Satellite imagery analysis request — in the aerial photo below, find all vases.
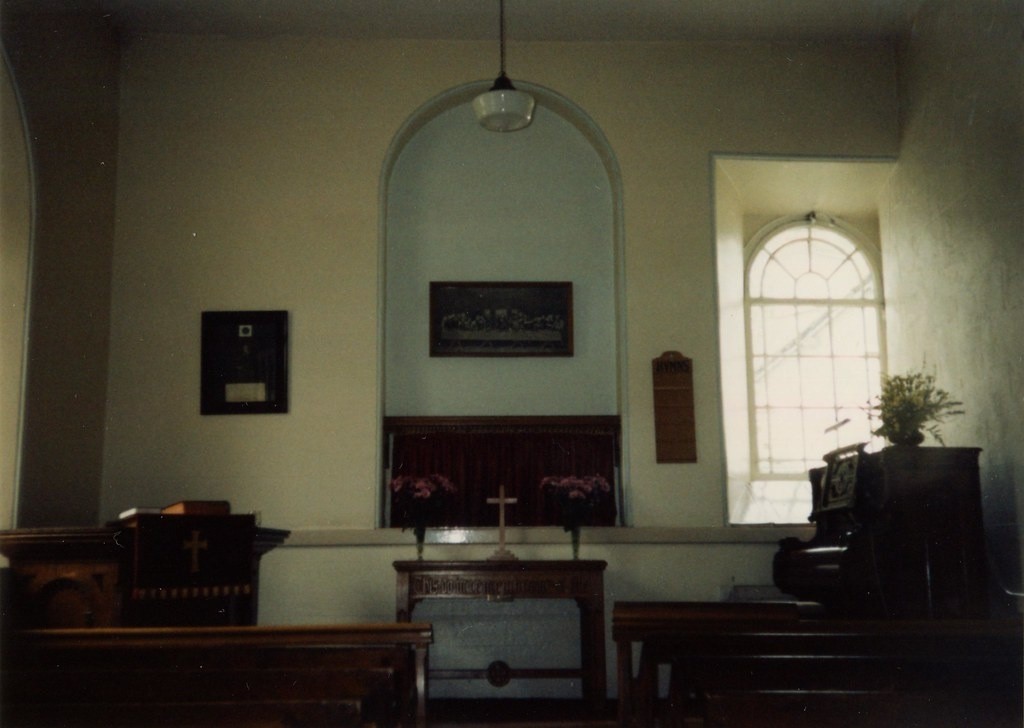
[416,527,425,560]
[571,526,581,559]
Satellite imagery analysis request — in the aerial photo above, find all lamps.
[473,0,535,134]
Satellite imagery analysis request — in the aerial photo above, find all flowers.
[387,473,463,541]
[539,474,609,548]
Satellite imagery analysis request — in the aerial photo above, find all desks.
[811,446,992,621]
[393,559,609,720]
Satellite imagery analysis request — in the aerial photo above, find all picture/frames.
[199,308,289,415]
[429,280,574,358]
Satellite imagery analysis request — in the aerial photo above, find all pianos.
[772,445,989,620]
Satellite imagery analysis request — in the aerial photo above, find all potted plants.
[863,370,966,447]
